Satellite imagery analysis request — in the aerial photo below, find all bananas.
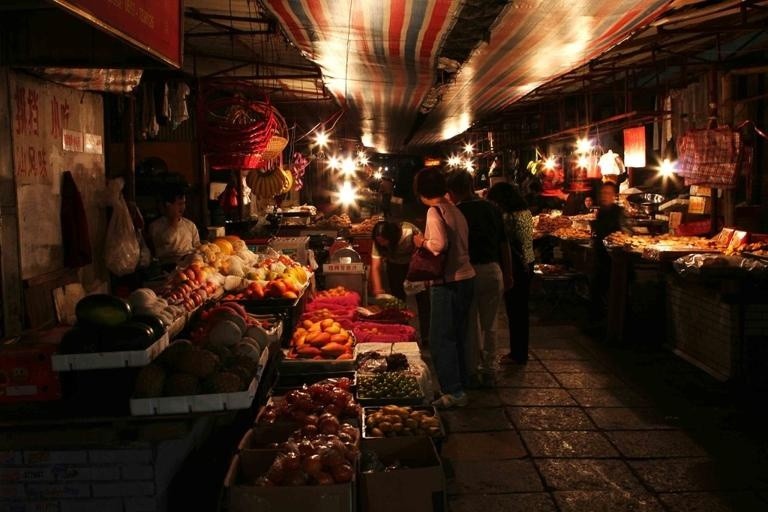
[246,167,291,200]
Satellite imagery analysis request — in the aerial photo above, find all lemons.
[251,378,441,488]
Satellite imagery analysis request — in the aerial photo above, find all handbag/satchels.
[676,117,742,187]
[405,205,445,282]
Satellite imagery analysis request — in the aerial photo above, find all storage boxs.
[222,405,448,512]
[0,326,69,404]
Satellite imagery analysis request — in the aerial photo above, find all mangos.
[289,317,352,359]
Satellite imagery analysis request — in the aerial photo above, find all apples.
[151,264,215,312]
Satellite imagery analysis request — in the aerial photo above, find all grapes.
[354,342,409,372]
[362,296,408,324]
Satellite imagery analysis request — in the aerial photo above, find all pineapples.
[133,338,256,398]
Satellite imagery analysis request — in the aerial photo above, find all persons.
[489,183,537,364]
[378,177,394,203]
[579,181,635,339]
[412,166,478,410]
[370,220,431,349]
[147,188,202,272]
[445,168,515,377]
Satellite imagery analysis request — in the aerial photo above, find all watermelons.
[57,292,164,351]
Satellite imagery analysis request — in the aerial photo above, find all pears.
[185,243,234,286]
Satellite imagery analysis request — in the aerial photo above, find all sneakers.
[499,353,519,366]
[431,390,468,408]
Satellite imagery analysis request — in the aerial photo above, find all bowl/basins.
[628,192,664,214]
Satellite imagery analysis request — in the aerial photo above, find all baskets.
[212,101,289,170]
